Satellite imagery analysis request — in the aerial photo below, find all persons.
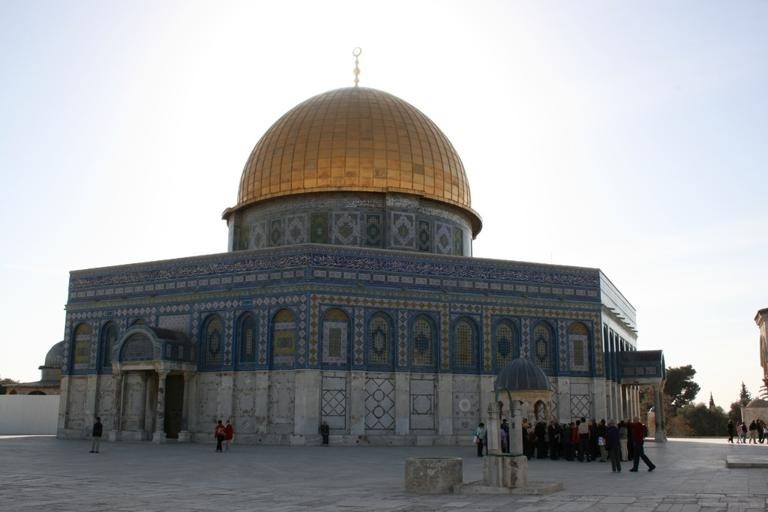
[224,420,233,452]
[214,419,226,453]
[89,416,102,453]
[318,421,330,447]
[727,418,768,444]
[475,416,657,473]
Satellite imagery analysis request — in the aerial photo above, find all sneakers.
[648,465,656,471]
[89,451,98,453]
[629,468,638,472]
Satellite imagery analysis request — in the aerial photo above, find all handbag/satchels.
[597,436,606,446]
[218,428,227,435]
[473,435,480,444]
